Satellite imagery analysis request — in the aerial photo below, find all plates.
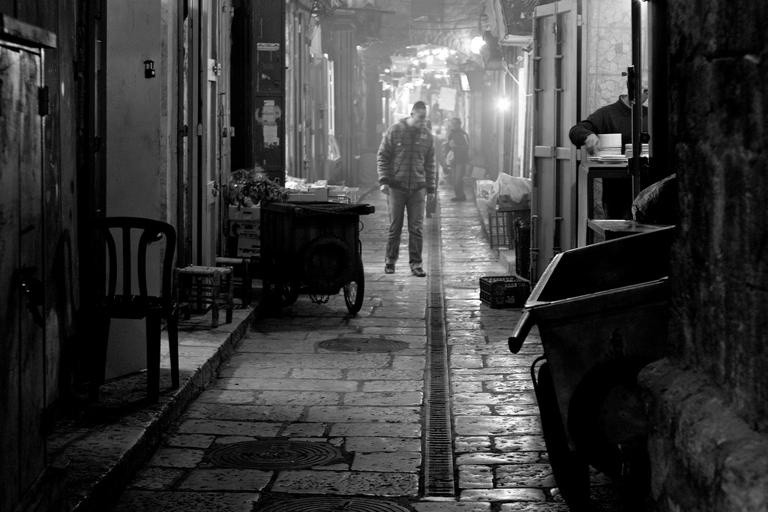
[587,143,649,164]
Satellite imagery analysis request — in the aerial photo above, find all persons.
[447,117,470,202]
[566,71,653,241]
[433,127,443,166]
[376,101,439,278]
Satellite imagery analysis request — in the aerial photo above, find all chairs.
[71,215,180,404]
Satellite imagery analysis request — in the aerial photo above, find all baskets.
[479,274,531,309]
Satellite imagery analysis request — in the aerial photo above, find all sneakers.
[384,264,396,274]
[412,268,426,277]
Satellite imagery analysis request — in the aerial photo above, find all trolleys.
[509,223,675,512]
[256,196,377,315]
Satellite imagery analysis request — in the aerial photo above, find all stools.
[172,257,253,329]
